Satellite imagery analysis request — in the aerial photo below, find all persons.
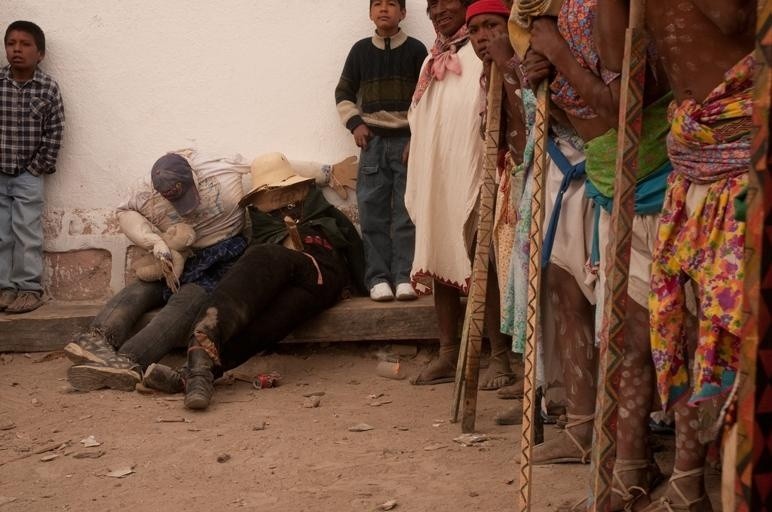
[143,151,361,409]
[405,2,772,512]
[333,0,434,290]
[64,147,361,391]
[0,20,65,313]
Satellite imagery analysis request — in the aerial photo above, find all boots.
[185,346,213,409]
[143,363,191,393]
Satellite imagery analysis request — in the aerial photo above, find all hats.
[237,151,314,207]
[151,153,200,216]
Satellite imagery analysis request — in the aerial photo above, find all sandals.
[492,400,714,510]
[408,343,526,398]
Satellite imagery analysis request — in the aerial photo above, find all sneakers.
[0,290,17,310]
[395,282,419,300]
[64,332,119,363]
[369,282,394,301]
[66,355,143,392]
[5,293,42,313]
